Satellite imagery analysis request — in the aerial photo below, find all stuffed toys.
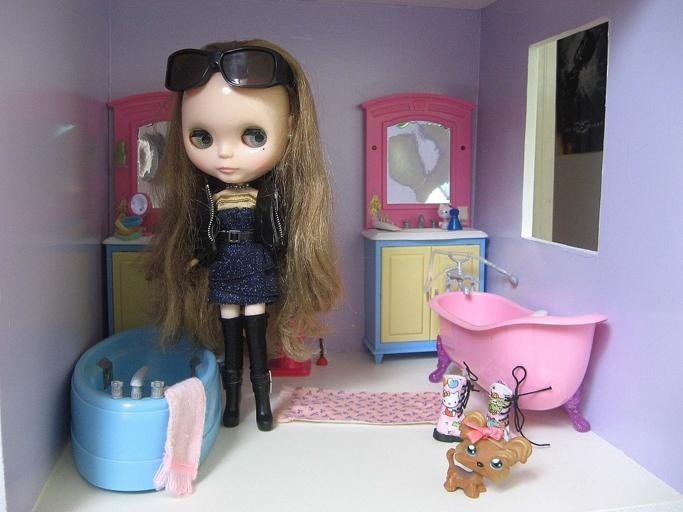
[445,409,531,497]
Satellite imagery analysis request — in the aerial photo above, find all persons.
[129,40,344,430]
